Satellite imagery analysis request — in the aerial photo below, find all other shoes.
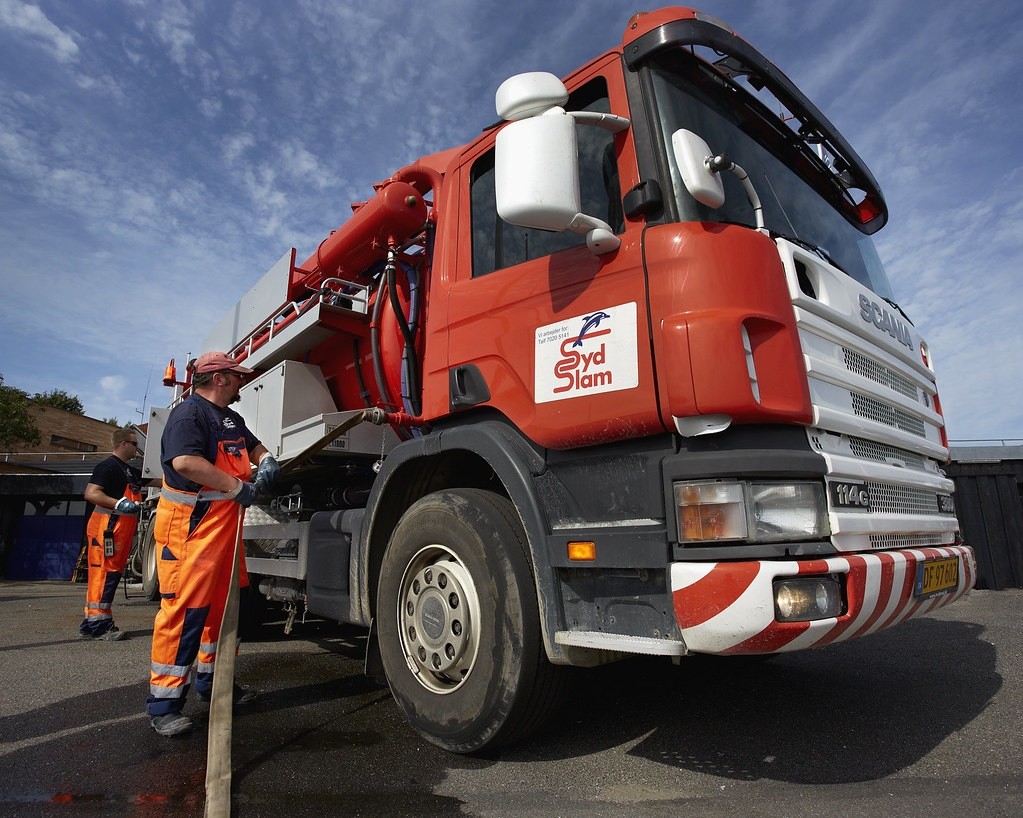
[151,711,193,735]
[197,682,262,705]
[93,625,125,641]
[78,624,92,637]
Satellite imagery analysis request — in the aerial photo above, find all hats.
[193,352,254,373]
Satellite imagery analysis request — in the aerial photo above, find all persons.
[79,427,165,644]
[147,351,284,736]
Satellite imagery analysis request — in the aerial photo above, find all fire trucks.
[129,3,981,757]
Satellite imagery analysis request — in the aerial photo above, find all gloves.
[254,452,281,495]
[222,476,259,508]
[114,497,141,514]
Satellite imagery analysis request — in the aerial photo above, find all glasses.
[212,370,244,380]
[118,441,137,447]
